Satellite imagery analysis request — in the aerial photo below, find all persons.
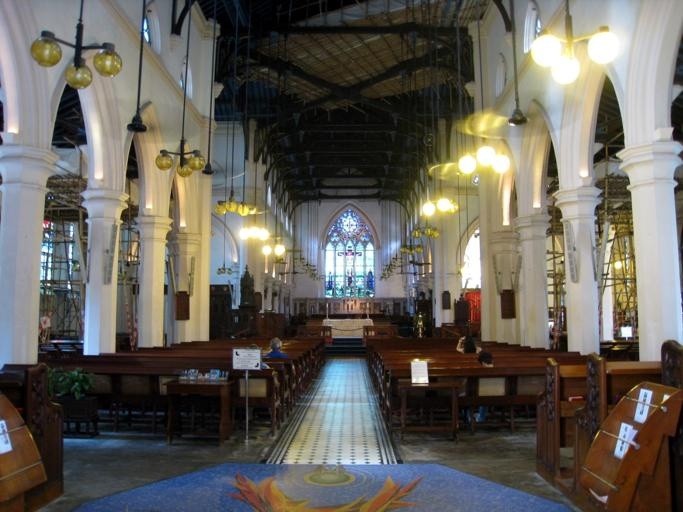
[455,335,483,356]
[264,337,288,359]
[245,344,270,369]
[476,351,495,425]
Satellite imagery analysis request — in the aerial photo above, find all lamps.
[25,1,325,286]
[377,2,622,278]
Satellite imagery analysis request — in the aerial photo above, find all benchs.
[0,322,337,451]
[358,322,683,506]
